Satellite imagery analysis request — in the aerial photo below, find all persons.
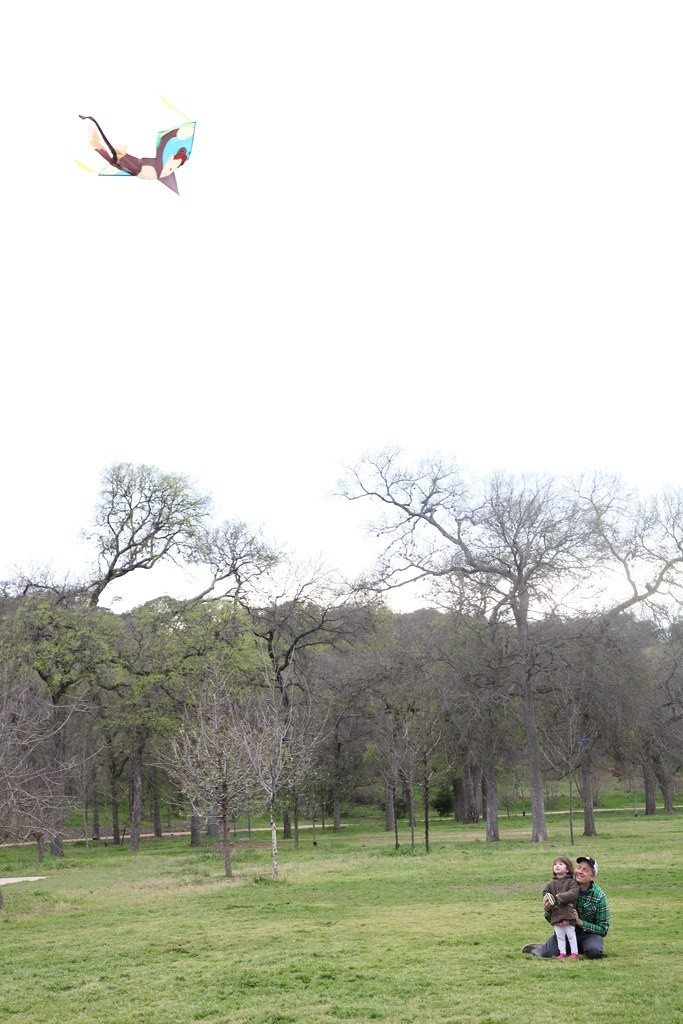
[542,857,579,960]
[522,857,610,959]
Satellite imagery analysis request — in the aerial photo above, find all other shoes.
[570,953,579,959]
[521,943,542,953]
[557,953,567,959]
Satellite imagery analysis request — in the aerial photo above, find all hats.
[576,856,598,876]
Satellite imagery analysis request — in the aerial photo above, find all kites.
[73,96,197,196]
